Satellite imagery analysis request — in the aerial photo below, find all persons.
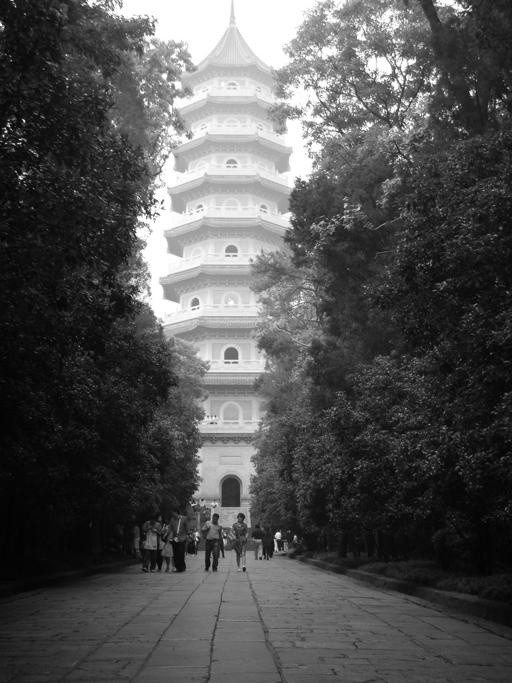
[128,510,297,573]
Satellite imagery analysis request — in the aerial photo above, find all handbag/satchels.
[161,544,173,558]
[227,535,236,548]
[143,532,158,551]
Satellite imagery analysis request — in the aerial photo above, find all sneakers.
[139,566,246,574]
[254,556,272,561]
